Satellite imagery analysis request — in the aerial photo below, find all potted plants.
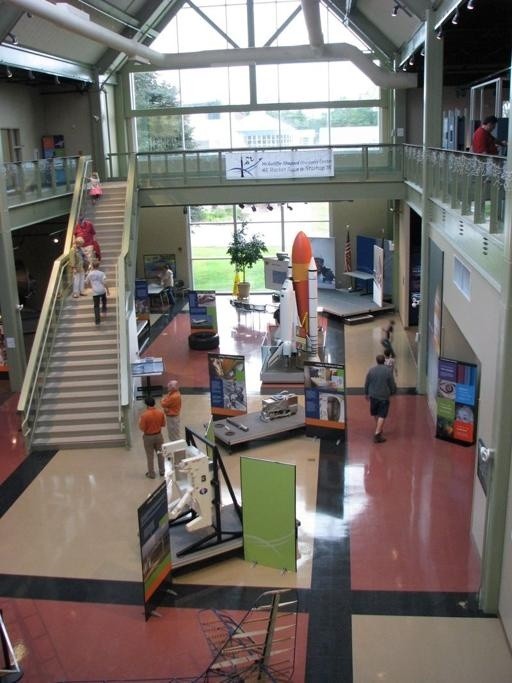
[226,220,269,298]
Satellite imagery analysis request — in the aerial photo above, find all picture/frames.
[477,438,492,498]
[143,254,176,278]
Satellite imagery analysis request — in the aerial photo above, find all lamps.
[182,206,187,215]
[287,202,293,211]
[420,48,425,57]
[55,76,60,85]
[452,7,461,25]
[409,53,416,66]
[466,0,476,10]
[6,66,12,78]
[27,70,35,80]
[238,204,244,208]
[403,62,408,71]
[391,4,400,16]
[266,203,273,211]
[9,33,18,44]
[436,26,445,40]
[251,204,257,212]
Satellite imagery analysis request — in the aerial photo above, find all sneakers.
[80,292,87,296]
[72,293,79,298]
[145,471,155,479]
[373,432,386,443]
[159,471,164,476]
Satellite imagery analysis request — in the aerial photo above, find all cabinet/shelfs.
[263,257,290,291]
[137,319,151,355]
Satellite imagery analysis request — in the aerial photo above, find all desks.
[341,270,373,296]
[132,372,163,400]
[231,299,279,329]
[148,283,169,312]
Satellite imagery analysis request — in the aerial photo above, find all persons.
[84,261,110,326]
[472,115,503,155]
[364,354,396,442]
[162,265,176,305]
[81,172,103,205]
[160,380,181,441]
[138,397,166,479]
[69,237,87,297]
[381,320,395,358]
[72,213,96,269]
[384,349,398,377]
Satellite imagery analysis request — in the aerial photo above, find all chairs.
[174,280,184,300]
